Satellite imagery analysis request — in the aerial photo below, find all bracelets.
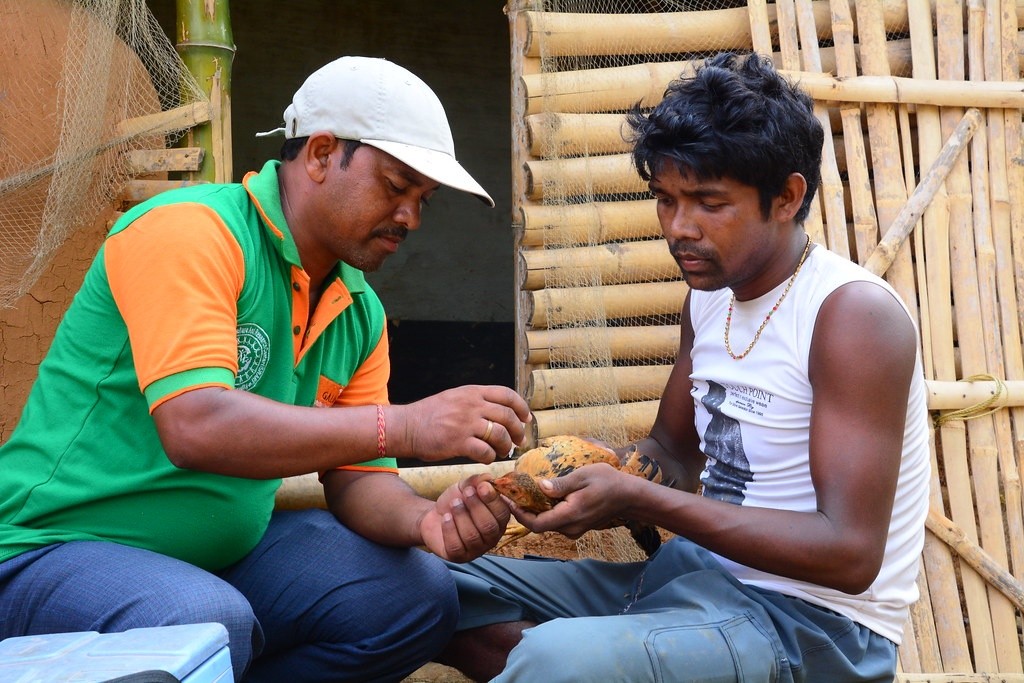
[377,404,386,459]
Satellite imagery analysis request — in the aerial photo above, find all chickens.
[480,434,681,558]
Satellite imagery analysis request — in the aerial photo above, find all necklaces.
[725,231,812,359]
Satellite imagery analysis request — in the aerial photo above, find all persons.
[426,51,931,683]
[0,56,533,683]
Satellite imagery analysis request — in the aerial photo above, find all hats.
[255,56,495,208]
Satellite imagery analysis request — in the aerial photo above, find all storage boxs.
[0,622,234,683]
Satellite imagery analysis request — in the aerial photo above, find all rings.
[482,420,493,441]
